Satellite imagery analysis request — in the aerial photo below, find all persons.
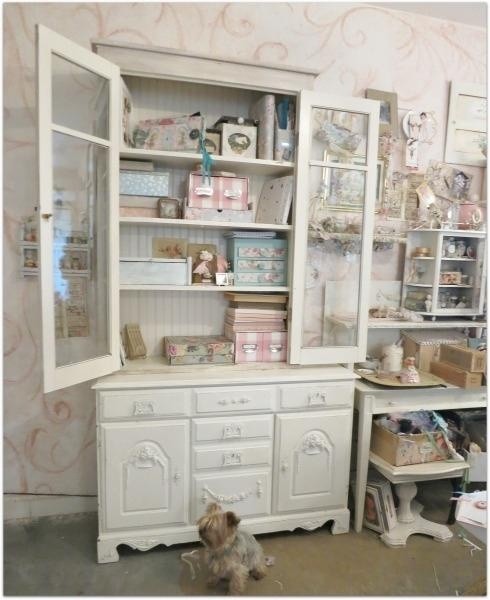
[222,291,288,332]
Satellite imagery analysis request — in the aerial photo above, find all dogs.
[192,502,268,596]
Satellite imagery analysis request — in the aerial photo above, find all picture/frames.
[20,221,41,277]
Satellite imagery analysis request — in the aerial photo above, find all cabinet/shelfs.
[34,23,383,393]
[353,88,489,548]
[95,368,354,564]
